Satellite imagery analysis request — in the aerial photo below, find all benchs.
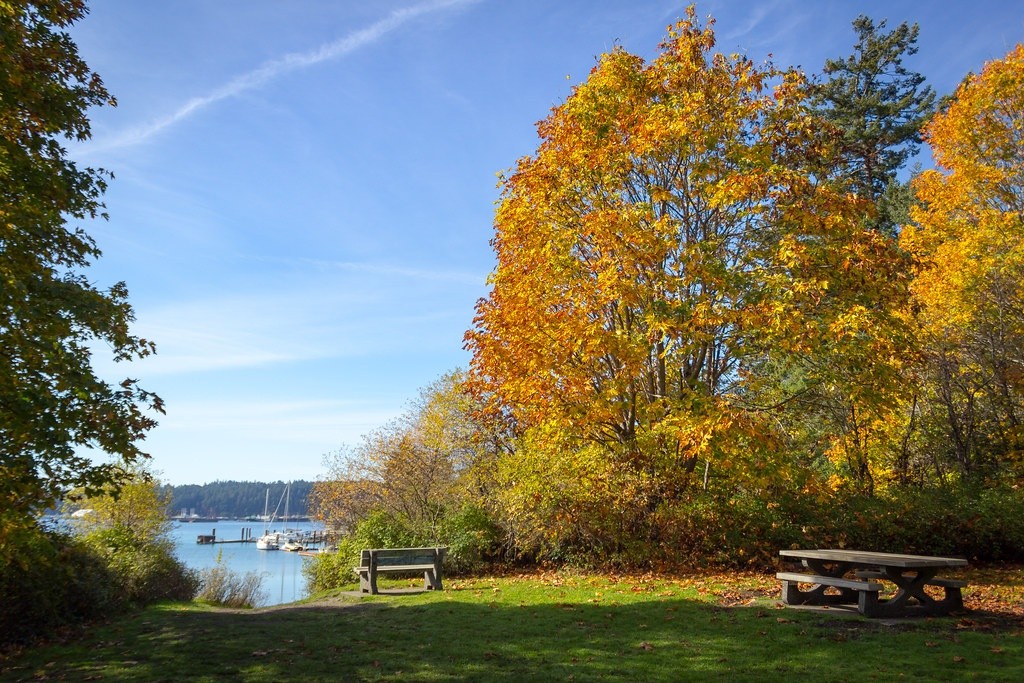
[855,571,967,608]
[354,548,447,595]
[776,572,884,615]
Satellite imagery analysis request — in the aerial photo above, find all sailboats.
[256,481,341,558]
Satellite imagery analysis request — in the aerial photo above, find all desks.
[780,549,969,617]
